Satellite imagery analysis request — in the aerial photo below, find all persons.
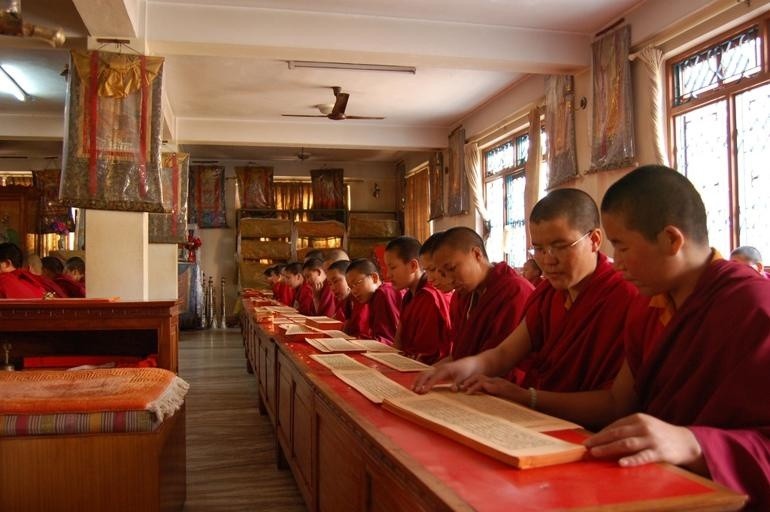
[263,165,770,512]
[0,242,85,298]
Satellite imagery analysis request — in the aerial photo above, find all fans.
[265,146,348,170]
[277,84,385,122]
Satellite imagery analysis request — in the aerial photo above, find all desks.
[1,292,188,380]
[174,258,209,332]
[235,285,757,511]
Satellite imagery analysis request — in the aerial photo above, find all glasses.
[347,275,368,292]
[526,230,593,261]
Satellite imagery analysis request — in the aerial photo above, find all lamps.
[0,65,42,106]
[284,60,418,78]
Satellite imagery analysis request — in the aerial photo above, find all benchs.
[1,364,189,511]
